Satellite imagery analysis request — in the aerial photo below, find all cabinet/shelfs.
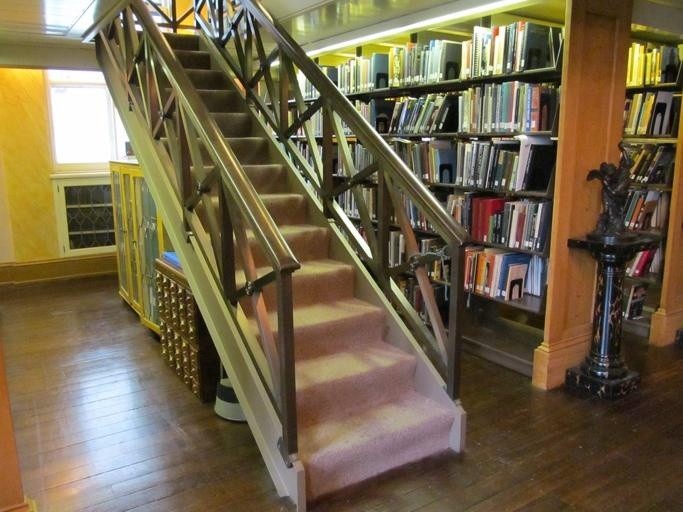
[110,159,164,336]
[155,251,221,401]
[620,58,683,348]
[254,0,632,392]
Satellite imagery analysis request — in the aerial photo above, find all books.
[385,22,566,319]
[605,36,683,293]
[289,53,388,250]
